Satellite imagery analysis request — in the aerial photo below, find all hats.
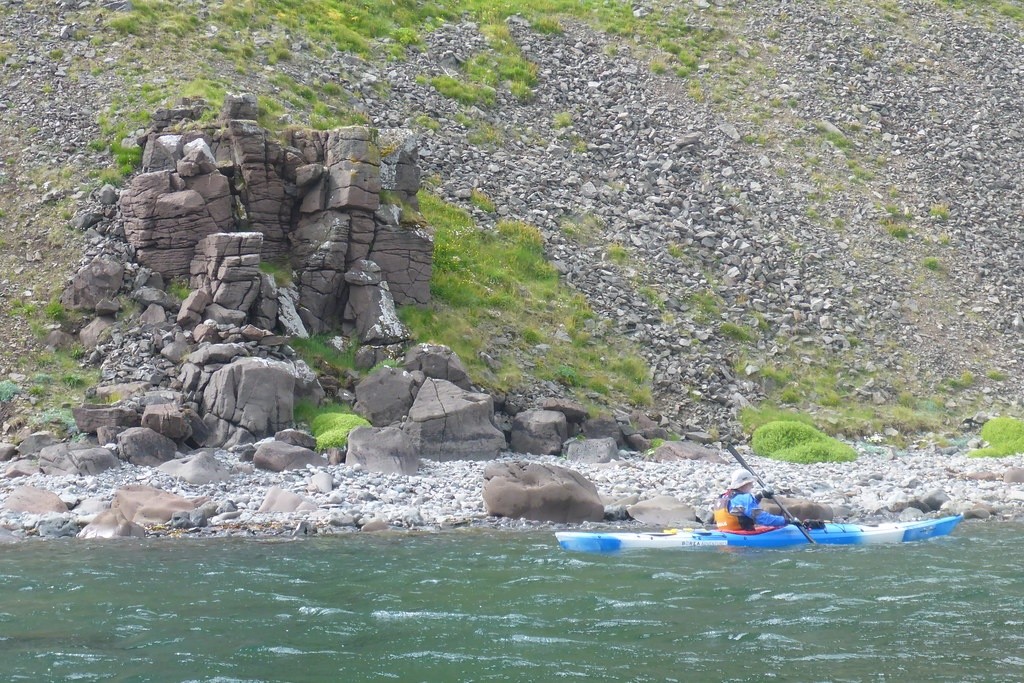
[727,469,758,491]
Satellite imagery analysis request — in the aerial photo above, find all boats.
[552,511,966,554]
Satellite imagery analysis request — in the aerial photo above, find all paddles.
[726,445,819,545]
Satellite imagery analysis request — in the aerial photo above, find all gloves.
[785,516,803,526]
[755,486,774,501]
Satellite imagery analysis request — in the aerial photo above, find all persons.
[714,469,802,531]
